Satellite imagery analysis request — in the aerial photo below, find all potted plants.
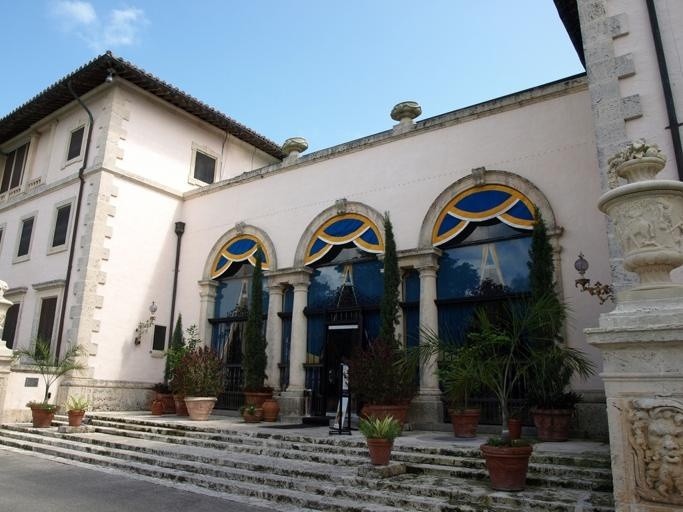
[245,238,275,418]
[416,284,600,492]
[347,208,413,436]
[238,401,265,425]
[62,393,89,427]
[356,411,402,466]
[153,382,176,412]
[442,358,484,438]
[182,345,228,420]
[9,335,90,428]
[521,346,599,443]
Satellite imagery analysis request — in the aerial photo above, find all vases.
[149,398,166,416]
[262,398,280,422]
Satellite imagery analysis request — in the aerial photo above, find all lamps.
[570,247,618,304]
[134,297,157,346]
[164,312,189,415]
